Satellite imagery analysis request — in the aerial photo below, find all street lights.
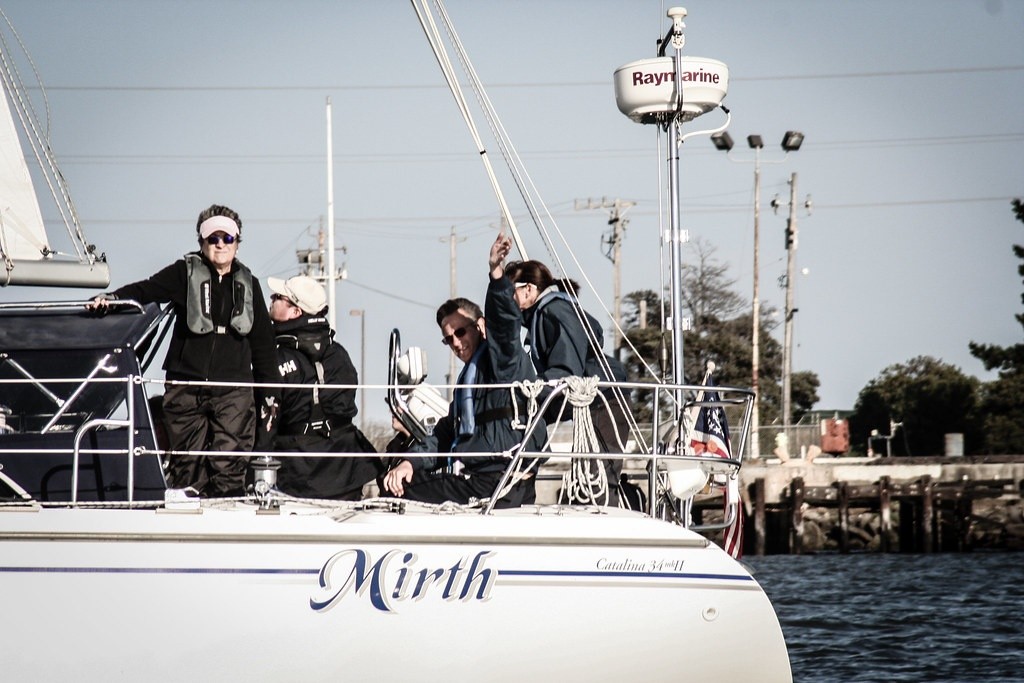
[710,130,803,464]
[350,309,365,435]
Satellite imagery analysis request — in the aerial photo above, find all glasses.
[270,293,299,308]
[442,321,477,345]
[207,234,237,244]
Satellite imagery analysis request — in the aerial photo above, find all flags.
[690,371,743,562]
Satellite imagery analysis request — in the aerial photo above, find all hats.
[198,215,239,239]
[268,276,328,316]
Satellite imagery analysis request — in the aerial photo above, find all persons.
[84,204,281,504]
[377,404,435,497]
[244,276,386,501]
[384,231,551,509]
[505,261,634,508]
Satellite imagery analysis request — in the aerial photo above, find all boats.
[0,0,793,683]
